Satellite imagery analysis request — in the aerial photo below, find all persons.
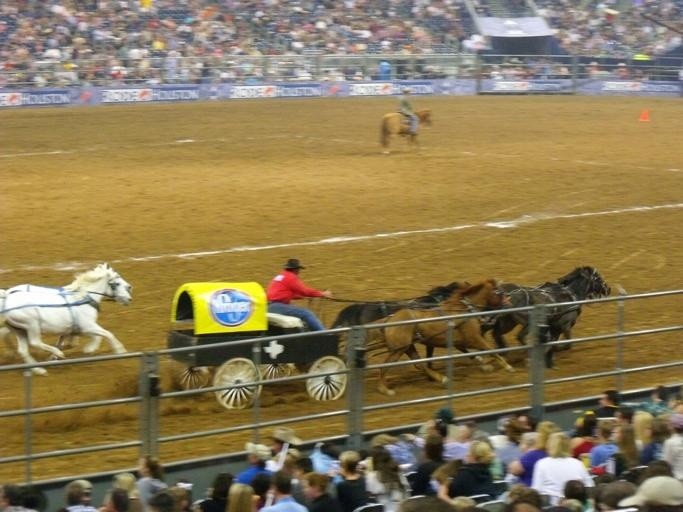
[0,387,682,512]
[267,258,333,332]
[401,87,419,135]
[1,0,682,88]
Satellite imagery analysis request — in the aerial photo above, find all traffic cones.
[638,109,649,122]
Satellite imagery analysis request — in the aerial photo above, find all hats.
[262,426,303,446]
[245,442,272,462]
[282,258,305,269]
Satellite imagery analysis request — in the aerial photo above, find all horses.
[380,108,433,155]
[330,265,483,382]
[0,261,132,378]
[482,264,611,371]
[376,277,516,396]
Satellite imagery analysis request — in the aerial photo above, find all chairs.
[352,464,650,511]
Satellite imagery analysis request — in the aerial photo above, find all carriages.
[167,265,611,410]
